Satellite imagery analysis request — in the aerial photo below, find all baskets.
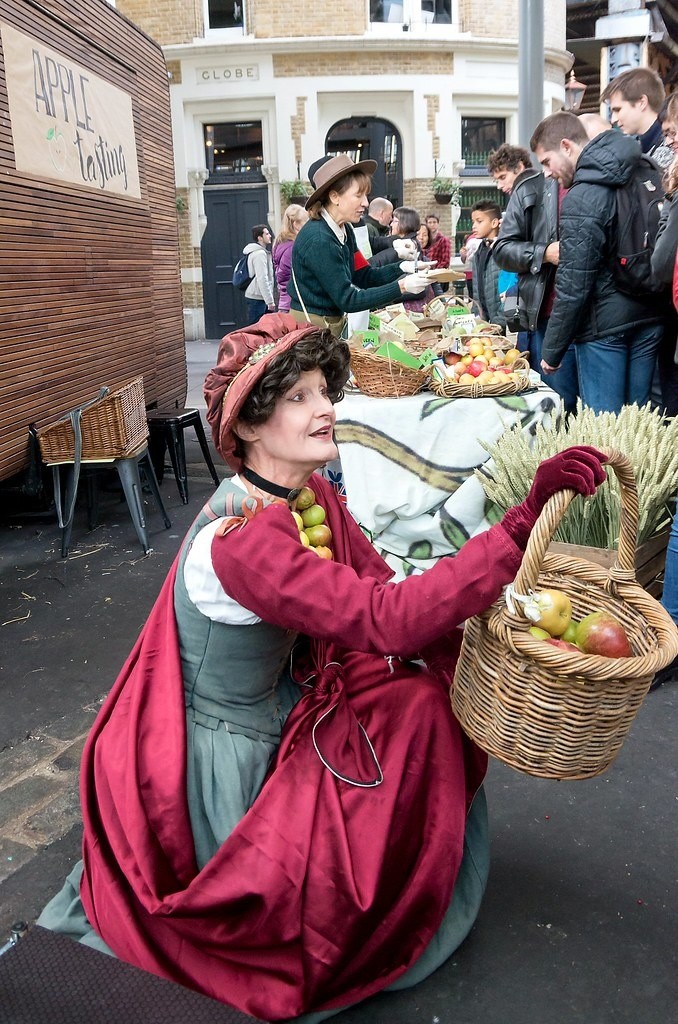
[35,377,151,460]
[346,332,429,398]
[447,443,677,782]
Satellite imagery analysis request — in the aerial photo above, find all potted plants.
[432,178,464,207]
[279,178,311,208]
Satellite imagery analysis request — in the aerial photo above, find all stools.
[61,441,170,558]
[147,409,220,506]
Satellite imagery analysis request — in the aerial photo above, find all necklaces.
[240,465,302,503]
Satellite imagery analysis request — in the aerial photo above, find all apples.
[529,589,633,657]
[289,485,333,562]
[444,337,523,385]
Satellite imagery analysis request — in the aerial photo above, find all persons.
[244,68,678,690]
[39,313,607,1024]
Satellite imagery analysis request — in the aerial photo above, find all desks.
[322,372,562,583]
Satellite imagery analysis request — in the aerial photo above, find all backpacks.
[612,152,673,301]
[232,250,256,291]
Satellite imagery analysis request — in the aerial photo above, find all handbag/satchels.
[503,284,530,333]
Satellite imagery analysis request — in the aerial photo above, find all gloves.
[497,444,609,550]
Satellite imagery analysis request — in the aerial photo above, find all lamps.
[562,70,587,111]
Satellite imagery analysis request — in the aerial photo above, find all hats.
[202,312,329,472]
[303,154,378,212]
